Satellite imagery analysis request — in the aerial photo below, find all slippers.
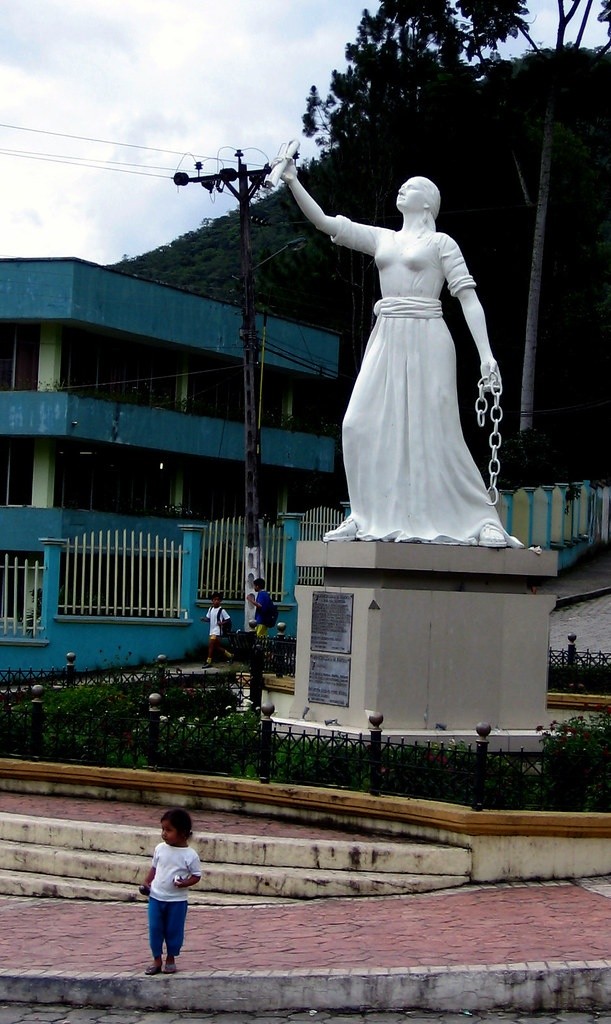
[145,965,161,975]
[164,963,176,973]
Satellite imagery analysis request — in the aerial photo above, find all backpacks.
[210,606,232,634]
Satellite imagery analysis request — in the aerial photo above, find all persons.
[246,578,274,662]
[143,808,202,975]
[270,152,526,549]
[198,594,234,669]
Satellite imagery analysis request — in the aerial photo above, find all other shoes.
[229,654,234,665]
[202,662,211,668]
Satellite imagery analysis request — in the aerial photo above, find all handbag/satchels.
[263,603,278,627]
